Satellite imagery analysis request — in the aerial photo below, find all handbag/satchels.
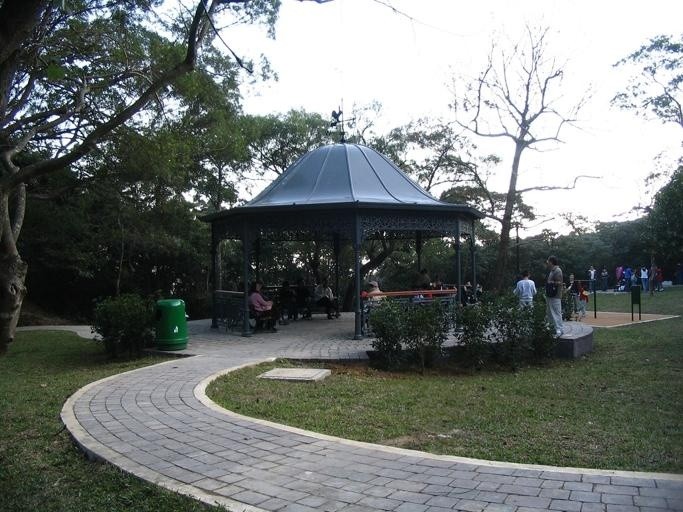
[545,283,558,297]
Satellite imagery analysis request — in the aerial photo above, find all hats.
[368,281,378,287]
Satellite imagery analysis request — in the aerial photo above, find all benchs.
[554,320,595,360]
[215,287,342,331]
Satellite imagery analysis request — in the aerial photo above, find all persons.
[249,280,274,323]
[413,281,483,318]
[542,255,563,340]
[511,270,537,327]
[361,281,386,326]
[584,262,663,290]
[568,273,589,318]
[280,275,335,321]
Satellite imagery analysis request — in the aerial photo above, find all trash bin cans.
[154,299,189,350]
